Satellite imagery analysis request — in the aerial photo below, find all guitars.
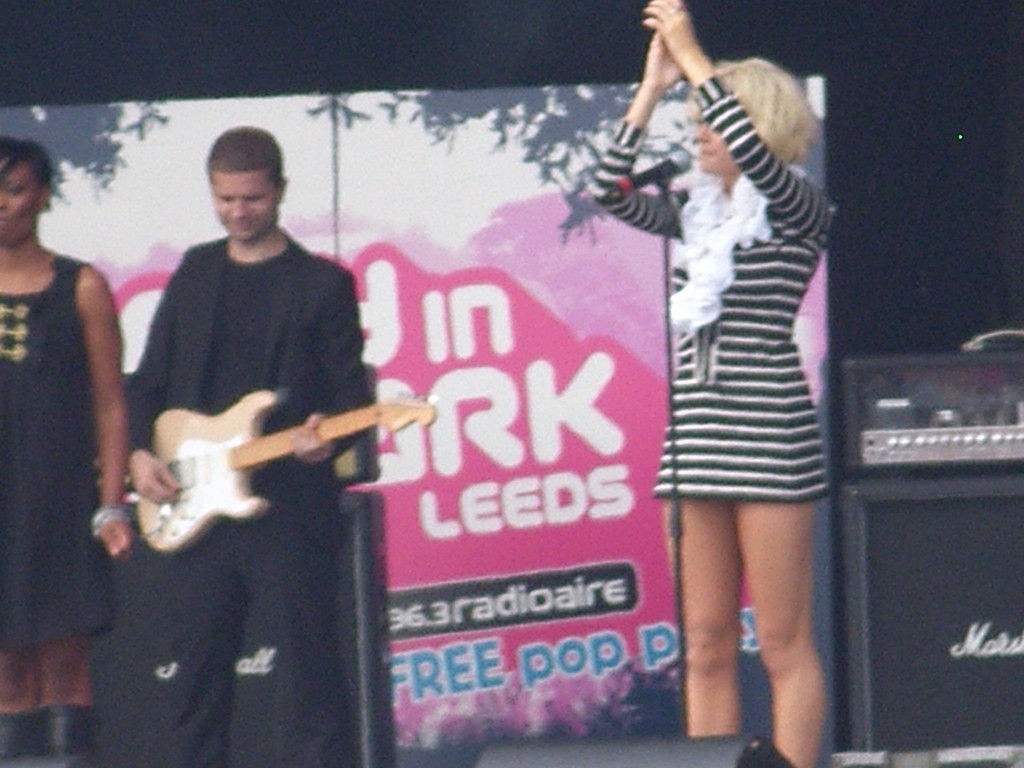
[136,389,439,553]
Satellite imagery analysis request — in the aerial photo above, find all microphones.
[599,152,690,201]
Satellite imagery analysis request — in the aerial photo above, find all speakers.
[844,479,1020,752]
[844,348,1023,473]
[91,492,394,768]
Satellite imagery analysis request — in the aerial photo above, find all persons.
[102,124,379,767]
[590,1,830,767]
[0,135,132,766]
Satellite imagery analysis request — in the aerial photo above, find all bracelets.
[90,505,131,538]
